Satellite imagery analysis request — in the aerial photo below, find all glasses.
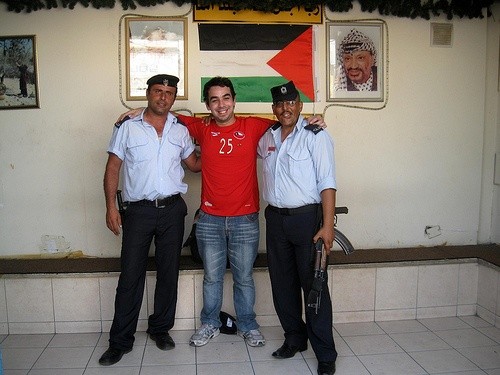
[272,99,298,107]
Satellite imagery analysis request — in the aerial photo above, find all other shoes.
[272,339,308,359]
[317,350,337,375]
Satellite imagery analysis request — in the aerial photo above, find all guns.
[306,201,355,316]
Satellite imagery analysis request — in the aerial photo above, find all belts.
[130,192,180,209]
[268,204,318,215]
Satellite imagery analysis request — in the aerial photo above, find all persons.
[202,80,338,375]
[99,74,215,366]
[117,77,327,347]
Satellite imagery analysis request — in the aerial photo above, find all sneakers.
[237,327,266,347]
[189,323,220,346]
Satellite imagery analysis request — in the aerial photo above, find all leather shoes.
[99,344,134,366]
[150,331,175,351]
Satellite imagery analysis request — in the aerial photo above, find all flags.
[197,22,321,103]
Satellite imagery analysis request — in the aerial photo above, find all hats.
[270,81,299,101]
[146,74,180,87]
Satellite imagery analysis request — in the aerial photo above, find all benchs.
[0,243,500,336]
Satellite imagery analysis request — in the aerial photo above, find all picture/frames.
[325,22,384,102]
[125,15,188,100]
[0,34,39,109]
